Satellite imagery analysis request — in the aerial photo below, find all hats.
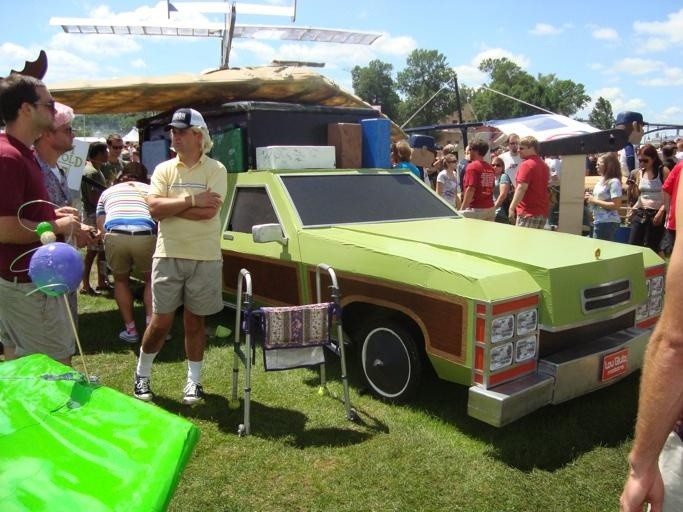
[163,108,204,132]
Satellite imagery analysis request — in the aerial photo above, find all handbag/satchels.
[503,183,515,212]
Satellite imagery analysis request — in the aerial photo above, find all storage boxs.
[357,118,391,169]
[256,146,336,170]
[327,122,362,169]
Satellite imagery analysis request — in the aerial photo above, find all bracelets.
[191,195,195,208]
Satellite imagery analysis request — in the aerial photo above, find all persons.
[133,107,228,407]
[389,133,683,260]
[0,73,78,368]
[33,101,101,331]
[80,133,172,343]
[619,159,683,512]
[615,111,649,176]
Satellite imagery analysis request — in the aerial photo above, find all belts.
[108,228,151,235]
[8,276,31,283]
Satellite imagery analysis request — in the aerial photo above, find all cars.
[95,164,668,430]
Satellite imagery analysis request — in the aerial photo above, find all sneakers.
[80,284,114,296]
[119,330,138,343]
[182,382,203,405]
[133,372,153,400]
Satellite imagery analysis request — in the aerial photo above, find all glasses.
[638,159,648,164]
[449,160,457,163]
[33,101,54,110]
[510,142,518,145]
[492,163,500,167]
[65,126,71,133]
[112,146,122,149]
